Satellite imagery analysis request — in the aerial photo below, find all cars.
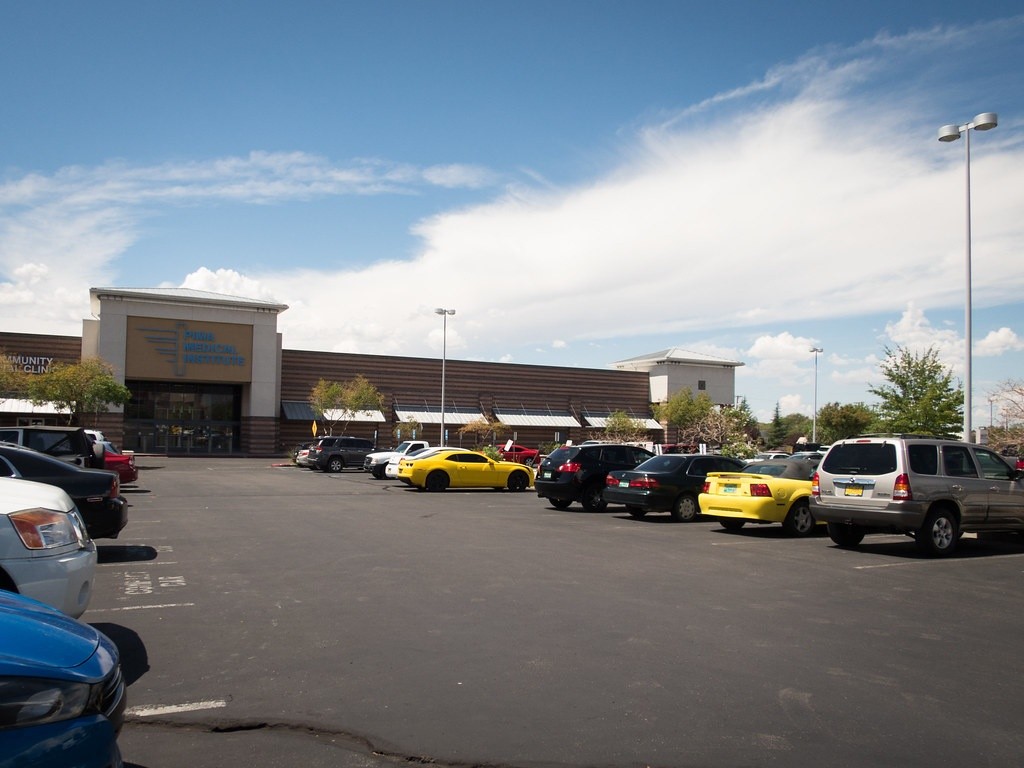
[0,473,97,618]
[480,445,541,467]
[87,435,137,486]
[581,440,700,454]
[744,445,831,464]
[85,429,106,440]
[385,447,472,480]
[0,591,127,768]
[95,441,121,455]
[293,439,320,471]
[0,440,128,542]
[603,454,747,522]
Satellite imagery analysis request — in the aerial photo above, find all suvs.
[307,437,394,472]
[0,425,104,468]
[808,433,1024,556]
[976,440,1024,472]
[532,446,659,511]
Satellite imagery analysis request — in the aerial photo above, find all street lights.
[434,308,456,447]
[809,347,823,444]
[937,112,999,469]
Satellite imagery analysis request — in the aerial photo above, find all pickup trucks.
[362,440,429,479]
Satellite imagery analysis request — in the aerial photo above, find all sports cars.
[697,458,828,537]
[398,450,535,492]
